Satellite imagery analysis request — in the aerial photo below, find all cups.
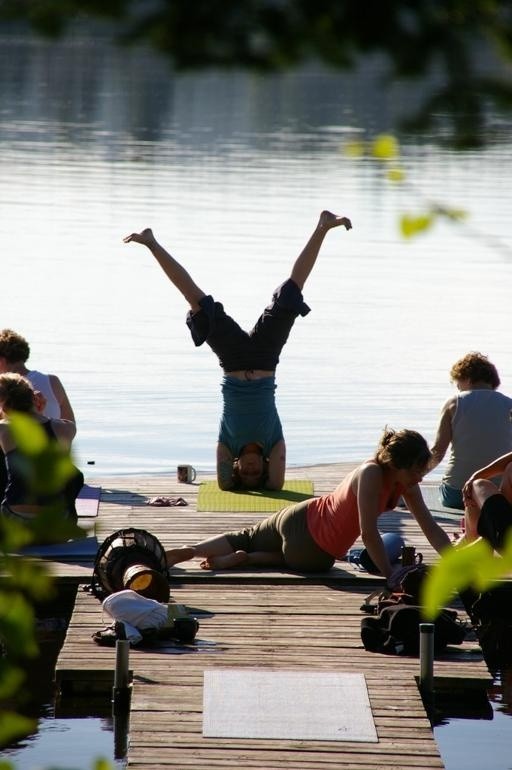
[172,616,199,643]
[402,546,422,566]
[177,464,196,483]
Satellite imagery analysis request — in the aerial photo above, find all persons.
[0,329,87,501]
[0,370,80,550]
[420,350,512,510]
[121,210,354,491]
[161,421,456,579]
[454,445,512,562]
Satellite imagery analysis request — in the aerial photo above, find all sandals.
[91,619,164,649]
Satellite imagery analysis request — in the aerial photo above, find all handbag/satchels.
[358,562,464,661]
